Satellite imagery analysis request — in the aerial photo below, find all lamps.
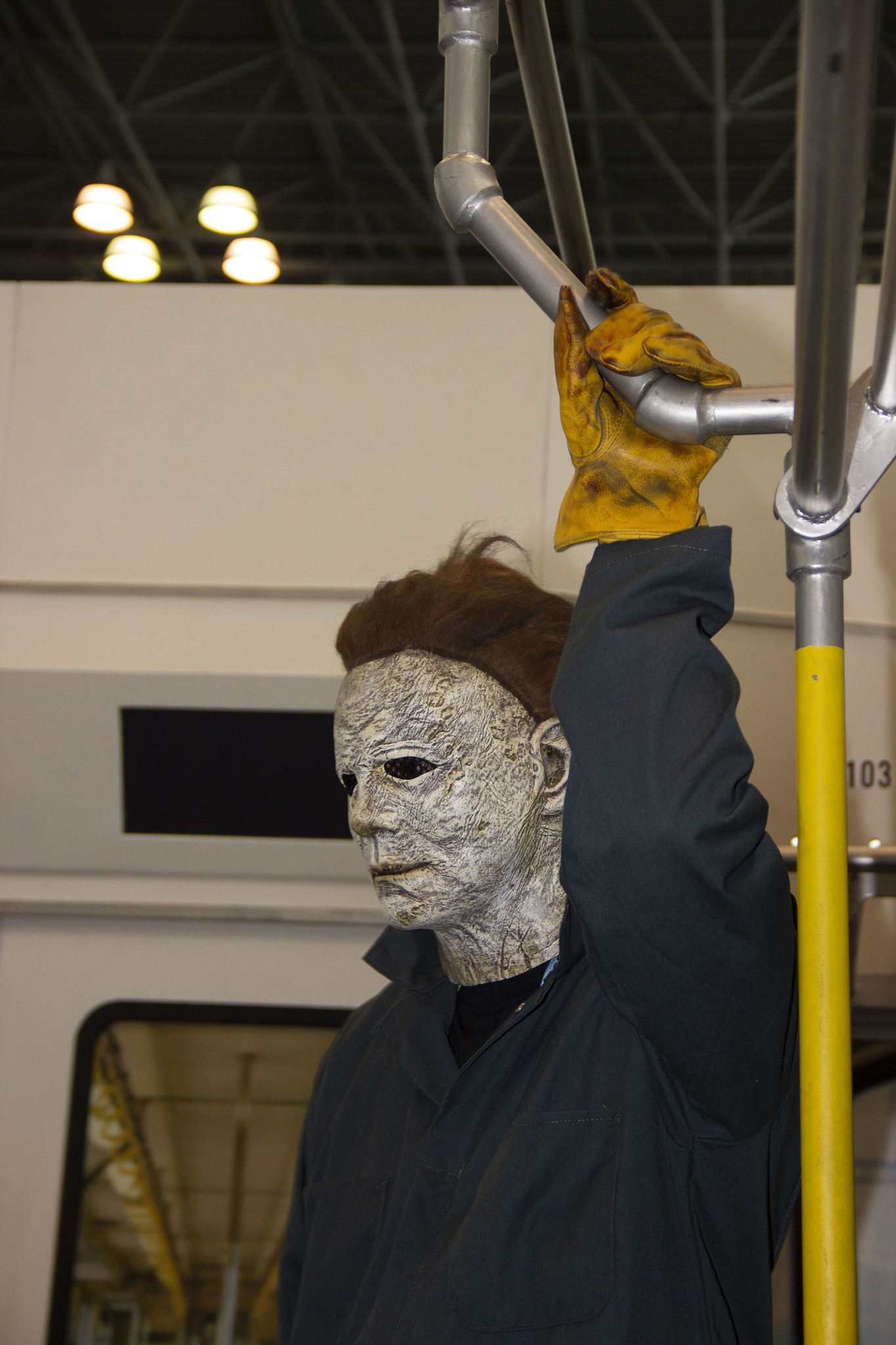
[193,153,285,286]
[72,153,162,285]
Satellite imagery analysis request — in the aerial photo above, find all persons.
[274,267,801,1345]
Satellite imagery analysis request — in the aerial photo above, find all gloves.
[551,267,741,553]
